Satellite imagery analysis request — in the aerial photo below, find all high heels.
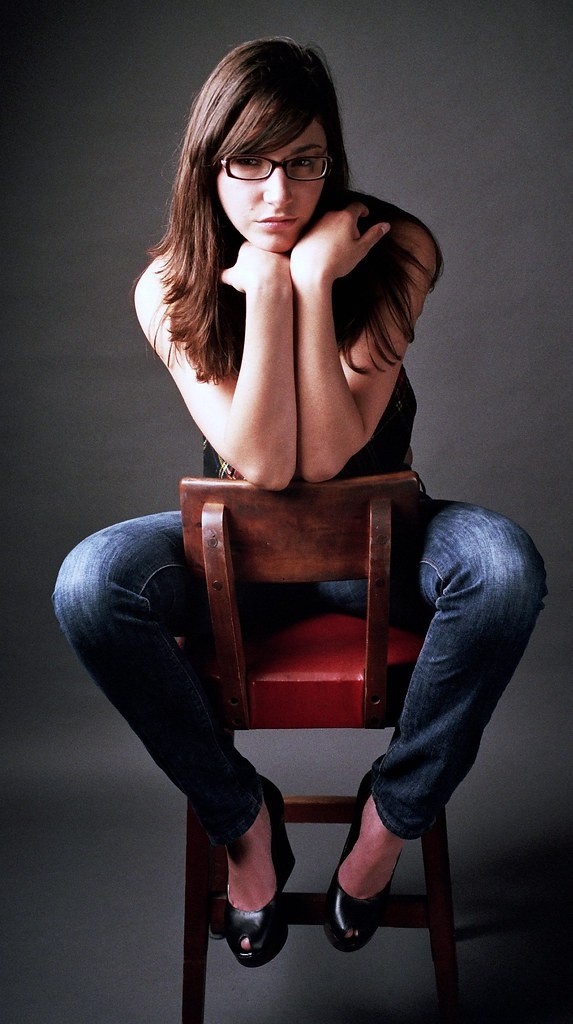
[322,769,403,952]
[224,773,295,967]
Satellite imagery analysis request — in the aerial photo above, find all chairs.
[179,469,459,1024]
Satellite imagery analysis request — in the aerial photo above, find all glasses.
[220,145,333,181]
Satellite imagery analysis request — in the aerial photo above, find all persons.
[51,36,548,969]
